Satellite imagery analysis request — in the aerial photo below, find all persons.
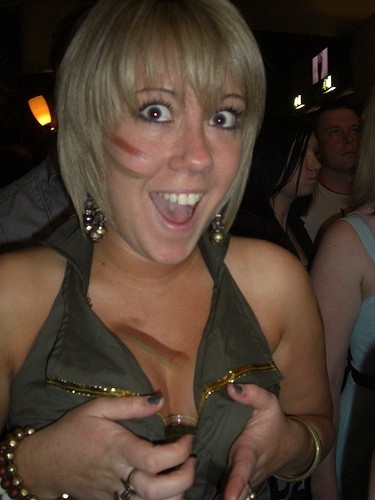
[228,116,323,273]
[1,7,97,252]
[288,100,363,248]
[0,0,339,500]
[309,88,375,498]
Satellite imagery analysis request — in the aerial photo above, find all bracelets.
[274,412,322,484]
[4,426,71,500]
[0,434,28,500]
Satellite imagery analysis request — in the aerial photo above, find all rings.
[245,482,257,500]
[115,467,141,500]
